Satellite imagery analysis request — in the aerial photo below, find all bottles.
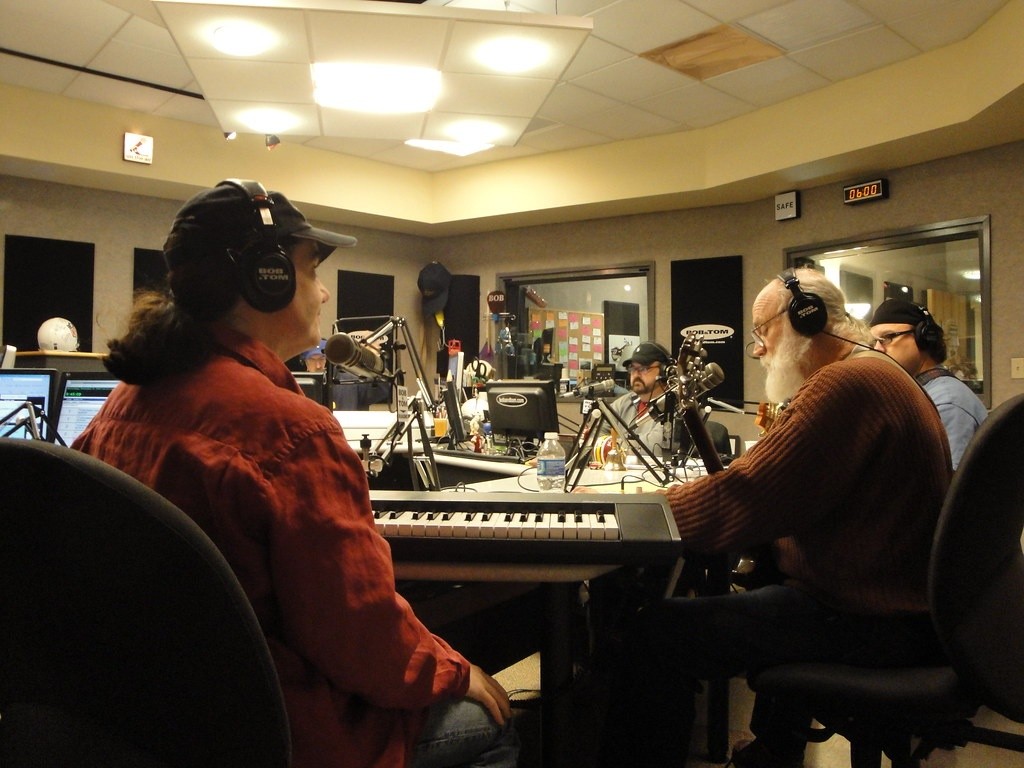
[536,433,565,494]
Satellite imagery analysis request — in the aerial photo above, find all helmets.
[36,318,78,352]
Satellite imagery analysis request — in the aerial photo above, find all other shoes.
[731,739,754,768]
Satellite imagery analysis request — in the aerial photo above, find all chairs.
[730,392,1023,768]
[0,436,293,767]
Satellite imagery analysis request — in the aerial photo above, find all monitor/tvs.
[48,372,122,448]
[485,380,560,454]
[442,380,467,450]
[290,371,333,415]
[0,368,58,443]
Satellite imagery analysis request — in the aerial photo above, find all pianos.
[369,485,684,560]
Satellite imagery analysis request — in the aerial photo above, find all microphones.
[324,332,392,381]
[629,362,725,430]
[560,379,615,399]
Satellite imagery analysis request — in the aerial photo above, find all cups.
[433,418,448,436]
[744,441,760,450]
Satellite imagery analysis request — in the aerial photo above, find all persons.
[867,297,990,471]
[583,341,686,456]
[70,179,522,768]
[584,268,957,768]
[300,338,358,380]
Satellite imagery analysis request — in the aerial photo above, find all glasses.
[750,306,789,346]
[871,329,914,348]
[628,365,659,375]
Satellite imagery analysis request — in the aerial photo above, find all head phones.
[299,337,328,365]
[642,341,678,385]
[215,178,297,312]
[777,267,828,336]
[908,301,942,352]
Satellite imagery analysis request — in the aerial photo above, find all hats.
[622,342,670,367]
[868,298,934,327]
[162,183,357,262]
[417,261,451,313]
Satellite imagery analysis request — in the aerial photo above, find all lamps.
[223,131,236,141]
[265,134,279,150]
